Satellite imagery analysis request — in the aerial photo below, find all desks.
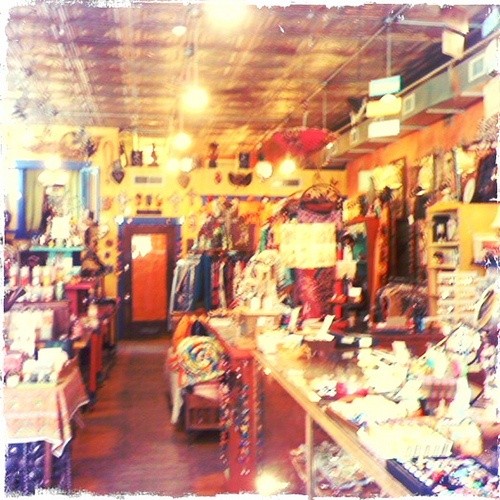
[206,317,257,493]
[252,349,412,498]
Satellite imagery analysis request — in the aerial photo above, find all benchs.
[163,309,219,439]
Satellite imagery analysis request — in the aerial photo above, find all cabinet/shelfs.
[4,232,123,498]
